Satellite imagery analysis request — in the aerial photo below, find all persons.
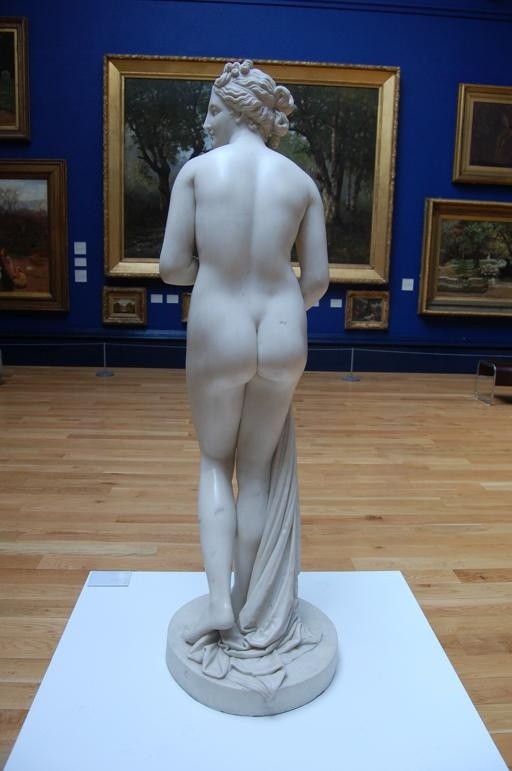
[160,55,334,650]
